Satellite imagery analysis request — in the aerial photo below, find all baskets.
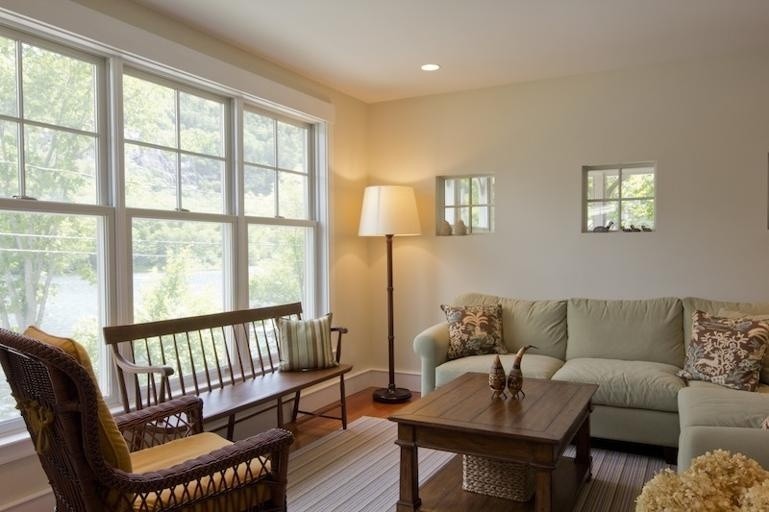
[462,453,537,502]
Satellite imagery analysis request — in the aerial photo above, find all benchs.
[104,302,354,457]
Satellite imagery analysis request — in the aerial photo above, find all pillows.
[276,312,336,372]
[442,304,509,357]
[677,309,768,392]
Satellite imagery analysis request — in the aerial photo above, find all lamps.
[357,186,420,402]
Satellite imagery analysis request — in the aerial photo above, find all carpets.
[285,416,676,511]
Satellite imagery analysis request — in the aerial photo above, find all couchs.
[408,293,766,485]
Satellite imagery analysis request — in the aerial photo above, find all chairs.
[0,322,292,511]
